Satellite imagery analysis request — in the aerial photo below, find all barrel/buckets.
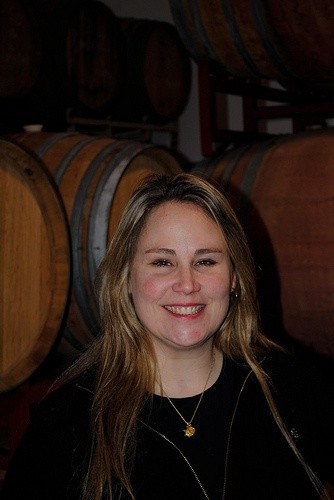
[0,1,334,135]
[184,122,334,352]
[0,140,71,393]
[0,132,186,368]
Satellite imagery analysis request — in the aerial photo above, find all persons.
[0,171,334,500]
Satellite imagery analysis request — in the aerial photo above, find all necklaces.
[155,344,217,438]
